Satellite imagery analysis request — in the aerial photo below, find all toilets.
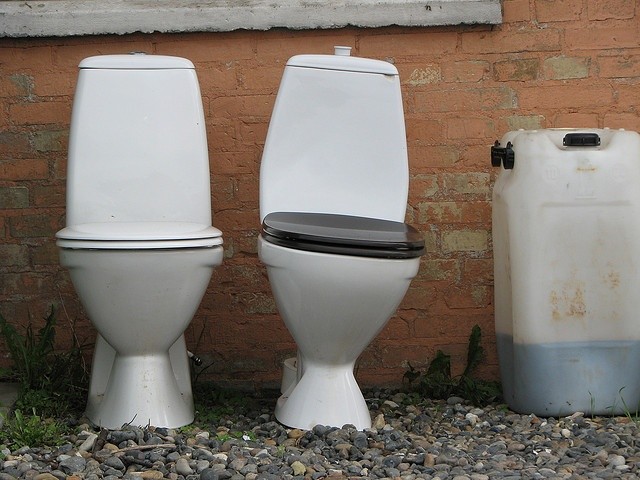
[54,52,224,431]
[258,45,426,432]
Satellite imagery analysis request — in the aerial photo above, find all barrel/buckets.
[491,126,640,417]
